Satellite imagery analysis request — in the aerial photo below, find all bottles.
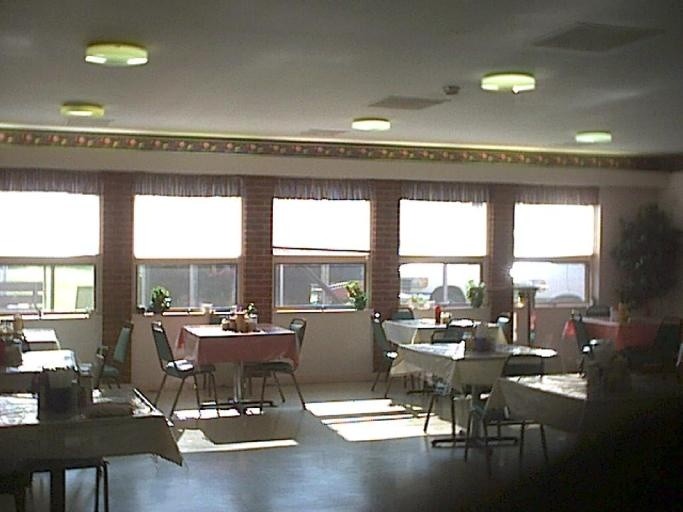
[0,311,26,367]
[609,301,633,323]
[582,339,602,381]
[465,319,497,353]
[434,305,448,325]
[222,314,256,332]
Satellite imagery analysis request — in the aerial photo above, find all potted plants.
[342,278,369,310]
[147,284,172,315]
[465,279,487,308]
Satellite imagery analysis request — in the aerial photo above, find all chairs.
[82,319,134,388]
[368,303,682,490]
[244,316,307,412]
[151,321,219,418]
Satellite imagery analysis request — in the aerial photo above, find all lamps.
[60,101,104,119]
[478,70,537,94]
[82,41,150,69]
[350,116,391,132]
[573,129,612,144]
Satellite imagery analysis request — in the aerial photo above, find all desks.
[1,317,109,511]
[176,322,299,416]
[1,385,183,512]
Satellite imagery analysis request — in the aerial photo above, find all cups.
[201,303,212,312]
[426,301,435,309]
[37,309,42,318]
